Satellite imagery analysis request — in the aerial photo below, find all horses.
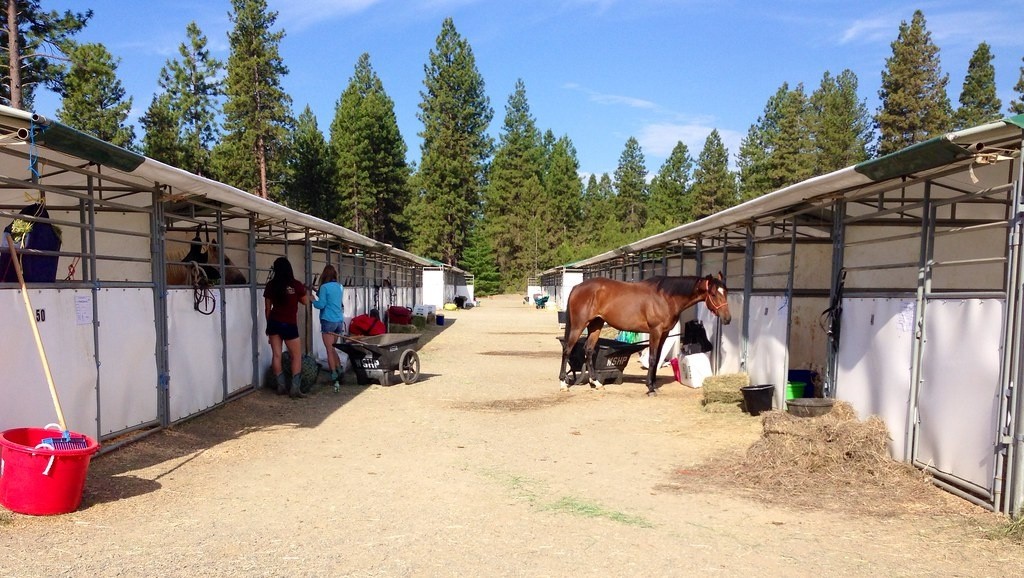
[558,270,732,398]
[165,237,247,286]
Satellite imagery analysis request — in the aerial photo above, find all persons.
[309,264,345,393]
[263,256,318,399]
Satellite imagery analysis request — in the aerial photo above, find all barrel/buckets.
[0,424,102,514]
[741,385,774,415]
[435,315,444,326]
[787,382,807,398]
[788,370,818,398]
[785,400,833,417]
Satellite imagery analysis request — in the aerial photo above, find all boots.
[274,372,289,395]
[289,373,307,399]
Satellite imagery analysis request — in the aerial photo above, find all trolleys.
[535,295,549,309]
[332,332,424,386]
[554,334,650,385]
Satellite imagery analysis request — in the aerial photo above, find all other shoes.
[332,370,339,382]
[337,367,345,377]
